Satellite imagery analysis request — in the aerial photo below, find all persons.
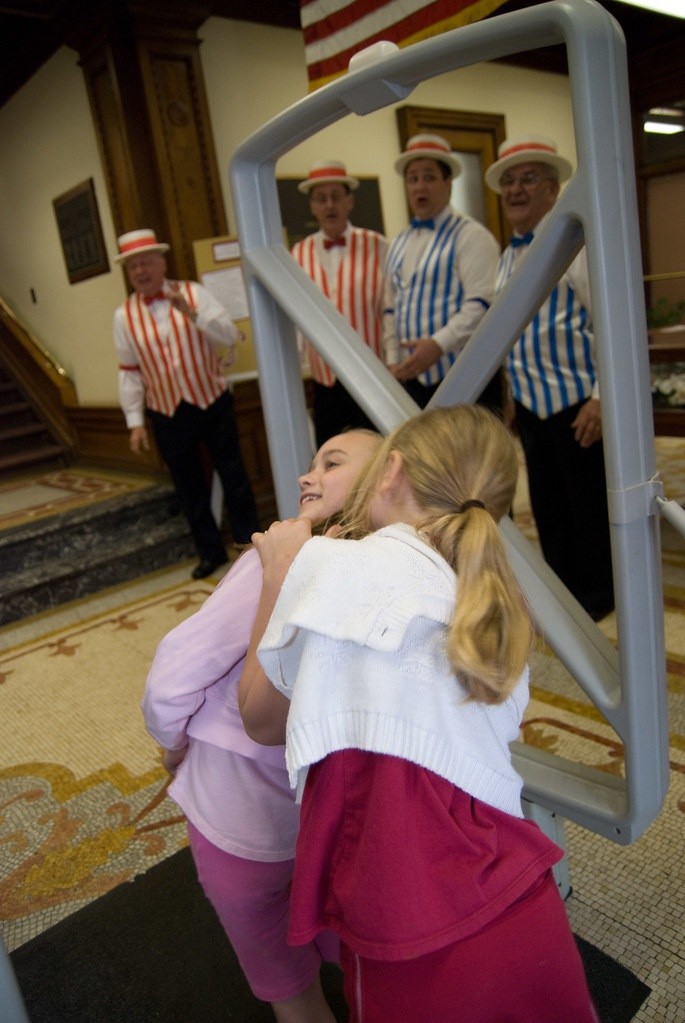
[142,429,384,1022]
[237,406,599,1022]
[290,159,390,450]
[486,136,615,622]
[112,230,258,579]
[384,134,513,523]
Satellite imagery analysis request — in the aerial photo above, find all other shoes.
[220,519,260,546]
[192,555,228,579]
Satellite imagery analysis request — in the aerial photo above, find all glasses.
[501,171,554,188]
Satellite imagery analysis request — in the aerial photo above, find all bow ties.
[145,292,164,305]
[410,218,434,229]
[511,228,534,247]
[323,237,346,250]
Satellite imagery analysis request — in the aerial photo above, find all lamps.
[641,112,685,134]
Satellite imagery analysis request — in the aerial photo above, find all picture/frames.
[51,176,111,286]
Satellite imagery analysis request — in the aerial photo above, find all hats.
[115,229,170,264]
[484,135,573,194]
[298,160,359,193]
[394,134,462,179]
